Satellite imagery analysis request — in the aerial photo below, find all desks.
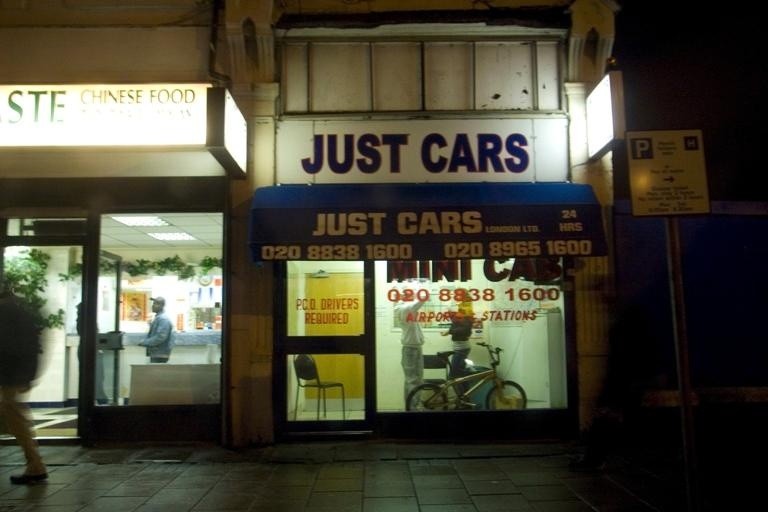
[129,364,221,406]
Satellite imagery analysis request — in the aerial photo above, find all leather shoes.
[10,471,47,483]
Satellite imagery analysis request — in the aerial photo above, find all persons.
[393,284,432,412]
[136,295,174,364]
[438,296,483,410]
[1,270,48,486]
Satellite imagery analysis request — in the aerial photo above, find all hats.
[149,296,165,305]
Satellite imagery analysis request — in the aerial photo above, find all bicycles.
[406,342,528,410]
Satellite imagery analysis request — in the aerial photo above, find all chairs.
[294,354,345,420]
[422,355,448,409]
